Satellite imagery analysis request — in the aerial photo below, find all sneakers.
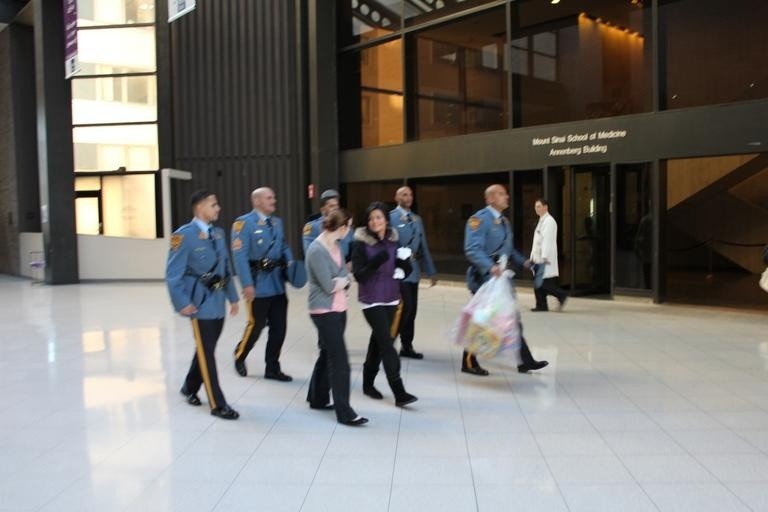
[396,393,417,406]
[363,387,382,399]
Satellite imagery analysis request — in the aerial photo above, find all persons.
[386,187,440,360]
[304,209,370,427]
[165,189,241,419]
[352,200,418,408]
[299,187,352,269]
[529,198,567,313]
[229,187,309,381]
[462,183,550,377]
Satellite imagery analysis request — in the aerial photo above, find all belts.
[410,251,419,259]
[188,272,231,291]
[250,258,287,274]
[489,255,499,262]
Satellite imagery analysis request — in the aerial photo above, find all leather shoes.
[342,415,368,426]
[532,308,548,311]
[518,360,548,373]
[462,366,489,376]
[211,405,239,419]
[180,388,201,405]
[399,350,424,360]
[264,371,293,381]
[312,403,335,410]
[235,358,247,376]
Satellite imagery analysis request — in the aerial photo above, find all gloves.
[368,249,390,272]
[396,258,413,278]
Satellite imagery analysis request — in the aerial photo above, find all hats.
[528,263,546,289]
[287,260,308,289]
